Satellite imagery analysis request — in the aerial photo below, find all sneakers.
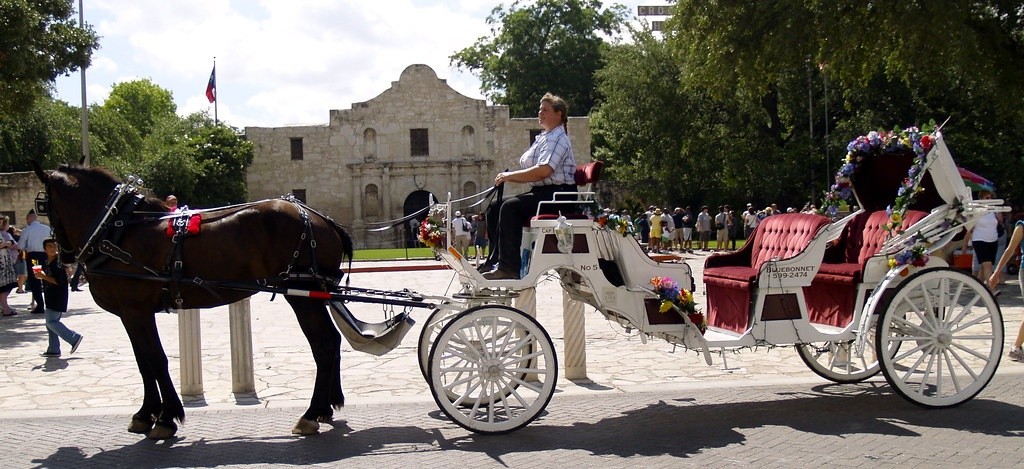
[1007,345,1024,363]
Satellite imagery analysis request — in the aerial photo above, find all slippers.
[3,311,17,317]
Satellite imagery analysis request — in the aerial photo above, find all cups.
[32,264,42,279]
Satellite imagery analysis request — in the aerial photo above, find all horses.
[30,158,353,439]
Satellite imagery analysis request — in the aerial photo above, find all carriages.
[35,128,1005,440]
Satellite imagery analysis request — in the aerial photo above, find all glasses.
[986,197,989,200]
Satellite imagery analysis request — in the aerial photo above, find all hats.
[654,208,663,214]
[166,195,177,202]
[455,210,461,216]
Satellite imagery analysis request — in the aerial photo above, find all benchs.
[812,210,927,285]
[602,222,695,293]
[701,212,831,288]
[524,160,604,227]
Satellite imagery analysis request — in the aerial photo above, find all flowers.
[647,276,708,336]
[415,209,445,250]
[814,119,969,275]
[578,194,636,239]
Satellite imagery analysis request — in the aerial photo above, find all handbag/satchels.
[462,218,469,231]
[993,290,1001,298]
[717,223,724,230]
[995,212,1005,238]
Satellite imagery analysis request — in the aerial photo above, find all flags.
[205,65,216,103]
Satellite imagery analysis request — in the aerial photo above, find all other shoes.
[70,335,84,354]
[40,351,61,357]
[482,267,520,280]
[31,309,44,314]
[479,265,494,273]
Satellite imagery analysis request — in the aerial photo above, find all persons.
[960,190,1024,362]
[408,210,490,261]
[479,92,577,279]
[0,208,86,356]
[587,202,819,255]
[166,194,177,210]
